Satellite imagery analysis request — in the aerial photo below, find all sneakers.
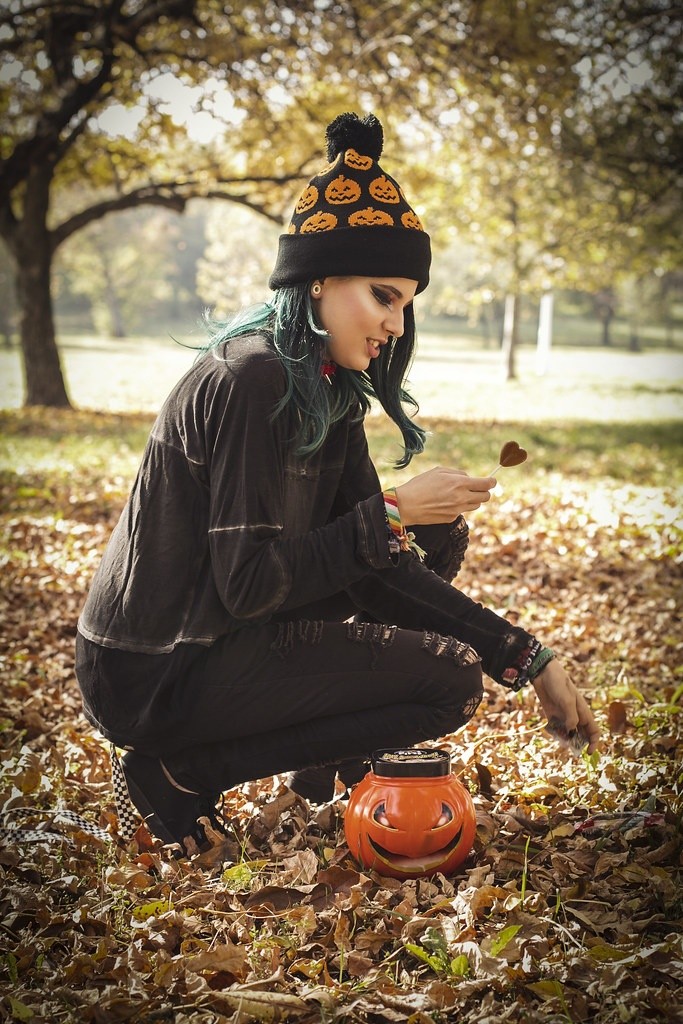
[289,760,373,806]
[120,746,233,862]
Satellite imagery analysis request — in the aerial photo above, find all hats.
[267,112,432,296]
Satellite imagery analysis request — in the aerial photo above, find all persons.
[72,110,604,867]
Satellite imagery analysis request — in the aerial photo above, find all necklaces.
[308,345,337,385]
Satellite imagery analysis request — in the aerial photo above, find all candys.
[487,441,528,477]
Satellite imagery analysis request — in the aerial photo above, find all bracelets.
[382,486,427,563]
[528,645,557,684]
